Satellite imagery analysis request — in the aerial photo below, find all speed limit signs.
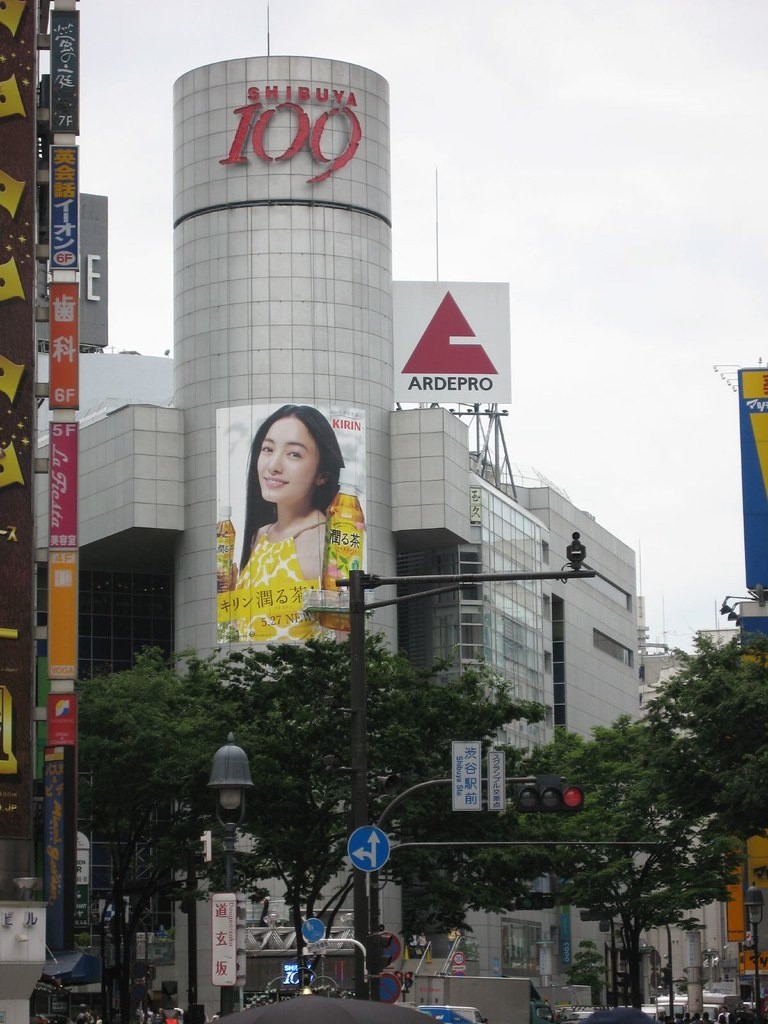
[453,951,464,965]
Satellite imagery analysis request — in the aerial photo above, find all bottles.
[216,506,235,592]
[320,467,364,631]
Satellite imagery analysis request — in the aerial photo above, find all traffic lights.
[514,779,585,814]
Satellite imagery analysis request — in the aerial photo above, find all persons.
[76,1004,103,1024]
[409,931,428,961]
[447,926,461,955]
[145,1007,222,1024]
[214,405,347,646]
[662,1006,768,1024]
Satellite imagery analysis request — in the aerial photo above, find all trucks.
[412,974,560,1024]
[560,993,742,1024]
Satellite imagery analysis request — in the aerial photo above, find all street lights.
[208,732,257,1018]
[742,881,764,1024]
